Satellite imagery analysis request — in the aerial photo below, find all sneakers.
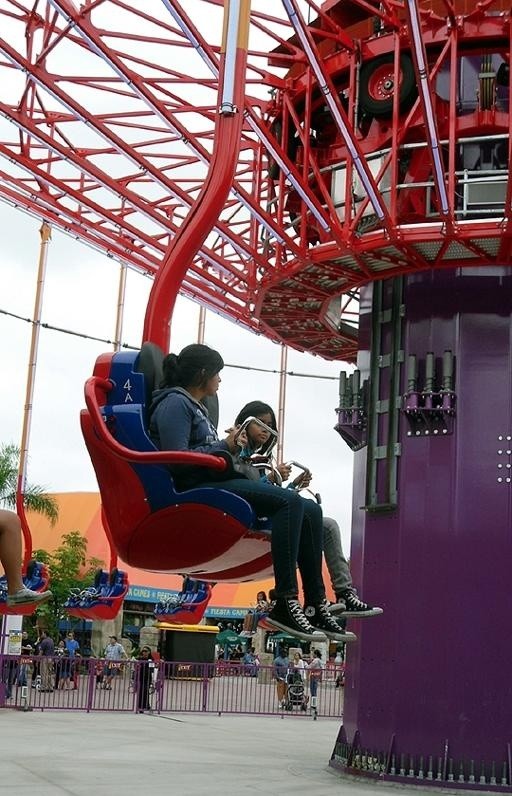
[266,588,384,643]
[6,584,53,607]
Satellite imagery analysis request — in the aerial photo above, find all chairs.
[79,340,324,584]
[243,608,283,635]
[153,575,216,625]
[62,568,130,621]
[0,559,52,616]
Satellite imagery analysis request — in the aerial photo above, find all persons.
[0,507,54,608]
[145,341,359,644]
[218,588,350,715]
[233,400,385,620]
[0,629,157,715]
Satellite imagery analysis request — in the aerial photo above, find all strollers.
[284,672,308,709]
[128,670,154,694]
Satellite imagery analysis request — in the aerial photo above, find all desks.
[224,659,242,663]
